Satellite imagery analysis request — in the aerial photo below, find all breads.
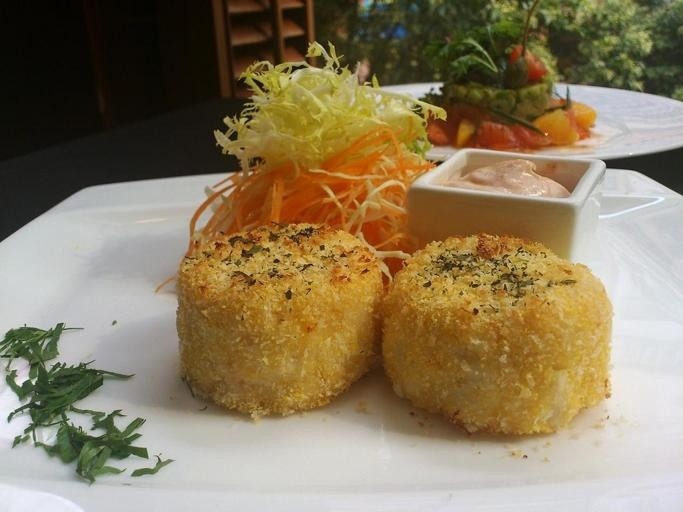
[380,233,613,437]
[177,223,384,416]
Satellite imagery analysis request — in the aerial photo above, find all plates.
[349,81,683,163]
[0,165,683,511]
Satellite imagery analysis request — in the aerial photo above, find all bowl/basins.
[409,145,607,266]
